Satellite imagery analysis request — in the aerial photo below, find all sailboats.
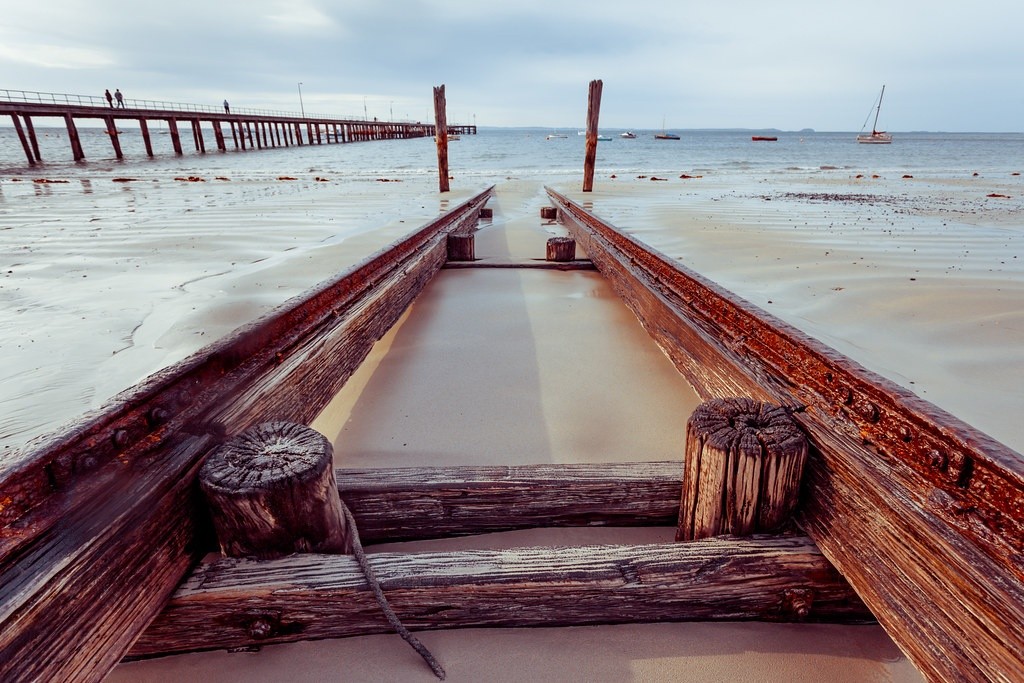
[856,84,894,144]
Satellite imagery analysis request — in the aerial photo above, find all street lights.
[297,82,431,125]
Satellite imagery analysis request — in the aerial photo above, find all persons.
[115,87,125,108]
[105,89,115,109]
[223,99,231,115]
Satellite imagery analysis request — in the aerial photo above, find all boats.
[654,132,681,139]
[752,135,777,141]
[433,130,637,143]
